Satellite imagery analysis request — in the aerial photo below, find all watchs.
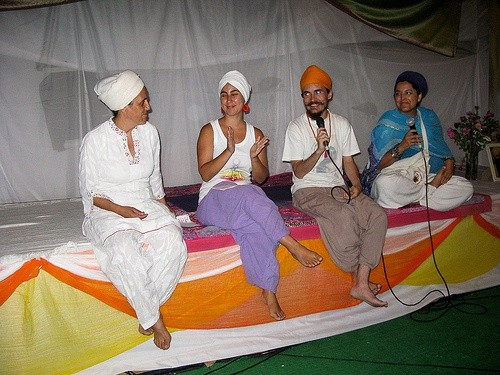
[389,149,398,158]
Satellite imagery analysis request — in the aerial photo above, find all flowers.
[447,106,500,152]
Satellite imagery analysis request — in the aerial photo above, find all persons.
[80,71,188,349]
[281,64,389,307]
[196,70,323,321]
[361,71,475,212]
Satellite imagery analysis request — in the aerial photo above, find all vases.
[465,151,478,178]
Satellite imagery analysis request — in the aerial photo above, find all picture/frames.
[485,142,500,182]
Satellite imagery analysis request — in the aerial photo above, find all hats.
[300,64,332,92]
[217,70,252,106]
[93,69,145,111]
[396,70,428,100]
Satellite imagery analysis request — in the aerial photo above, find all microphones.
[316,117,330,153]
[406,119,422,149]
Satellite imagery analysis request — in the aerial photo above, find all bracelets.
[393,144,404,155]
